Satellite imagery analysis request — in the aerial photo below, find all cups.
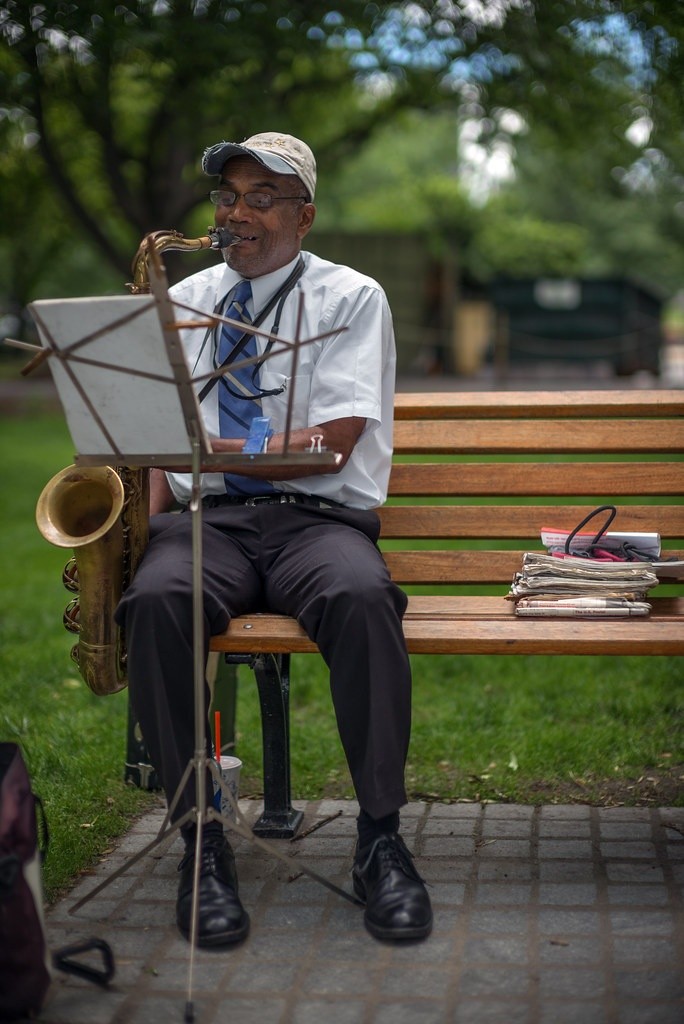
[213,756,241,831]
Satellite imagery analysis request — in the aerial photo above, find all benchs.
[130,387,684,841]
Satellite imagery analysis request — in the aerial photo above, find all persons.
[113,132,433,952]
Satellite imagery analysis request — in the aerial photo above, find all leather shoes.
[352,828,432,939]
[175,837,250,947]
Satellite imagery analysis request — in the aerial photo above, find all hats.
[201,132,317,203]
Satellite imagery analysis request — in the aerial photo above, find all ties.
[217,279,281,494]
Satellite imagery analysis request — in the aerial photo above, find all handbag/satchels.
[540,503,662,563]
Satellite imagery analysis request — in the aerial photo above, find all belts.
[182,491,343,511]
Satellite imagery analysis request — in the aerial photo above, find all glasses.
[204,189,308,208]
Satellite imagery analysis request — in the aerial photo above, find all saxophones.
[35,224,245,697]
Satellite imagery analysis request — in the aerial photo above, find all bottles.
[211,750,222,816]
